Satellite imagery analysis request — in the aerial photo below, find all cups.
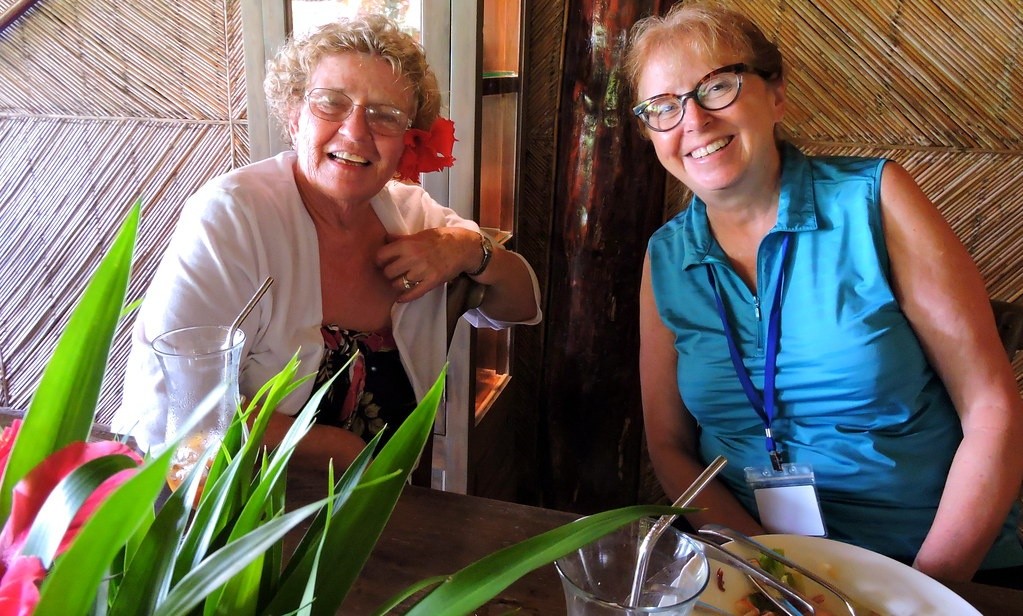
[554,515,709,616]
[149,324,246,510]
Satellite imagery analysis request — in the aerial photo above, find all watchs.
[466,232,493,277]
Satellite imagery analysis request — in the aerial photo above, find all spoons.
[678,523,882,616]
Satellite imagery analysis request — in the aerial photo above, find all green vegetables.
[759,545,796,589]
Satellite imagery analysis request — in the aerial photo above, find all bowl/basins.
[657,534,985,616]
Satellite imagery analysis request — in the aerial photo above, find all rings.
[402,275,411,289]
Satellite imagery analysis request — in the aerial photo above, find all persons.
[113,18,545,495]
[622,1,1023,594]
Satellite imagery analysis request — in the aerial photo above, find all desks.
[246,459,1022,616]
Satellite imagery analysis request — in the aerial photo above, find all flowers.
[0,195,714,616]
[393,115,459,184]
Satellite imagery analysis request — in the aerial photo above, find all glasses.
[633,62,770,132]
[303,88,413,138]
